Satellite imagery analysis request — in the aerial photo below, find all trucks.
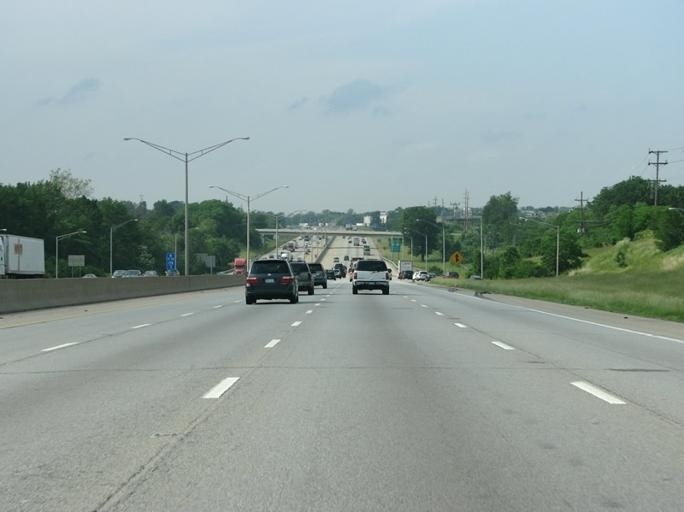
[232,258,247,276]
[0,233,47,279]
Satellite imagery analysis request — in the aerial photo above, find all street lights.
[122,135,250,278]
[436,216,484,281]
[107,217,138,278]
[54,229,87,279]
[519,216,561,277]
[274,208,305,260]
[208,183,288,278]
[414,218,447,277]
[402,226,430,274]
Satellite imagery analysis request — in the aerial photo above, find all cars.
[326,269,335,280]
[334,236,391,294]
[279,235,326,260]
[307,262,327,289]
[244,258,299,304]
[397,259,436,281]
[111,269,159,278]
[289,261,314,295]
[82,273,97,278]
